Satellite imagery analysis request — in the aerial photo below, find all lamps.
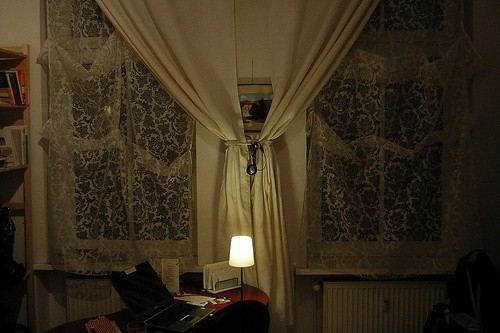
[228,236,254,301]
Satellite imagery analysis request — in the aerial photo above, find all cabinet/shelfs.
[0,44,36,333]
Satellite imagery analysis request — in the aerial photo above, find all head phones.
[246,141,258,175]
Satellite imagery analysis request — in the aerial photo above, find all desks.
[49,284,270,333]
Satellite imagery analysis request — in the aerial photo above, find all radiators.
[322,281,458,333]
[67,280,124,321]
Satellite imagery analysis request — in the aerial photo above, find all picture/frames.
[237,83,273,132]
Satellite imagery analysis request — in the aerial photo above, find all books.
[0,70,29,168]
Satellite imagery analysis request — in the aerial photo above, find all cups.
[127,321,147,333]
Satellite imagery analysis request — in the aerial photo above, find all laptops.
[109,260,215,333]
